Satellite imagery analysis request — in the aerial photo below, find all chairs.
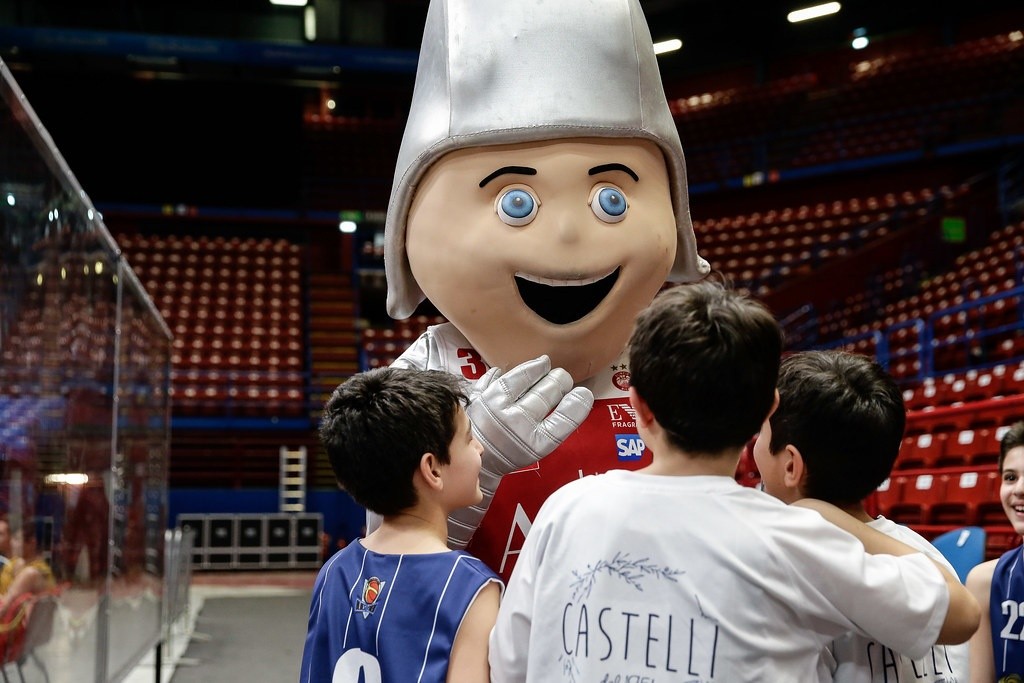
[0,583,74,683]
[0,10,1024,559]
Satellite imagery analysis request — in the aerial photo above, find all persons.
[365,0,765,584]
[0,518,54,618]
[299,367,505,683]
[965,425,1024,683]
[752,350,970,683]
[488,283,982,683]
